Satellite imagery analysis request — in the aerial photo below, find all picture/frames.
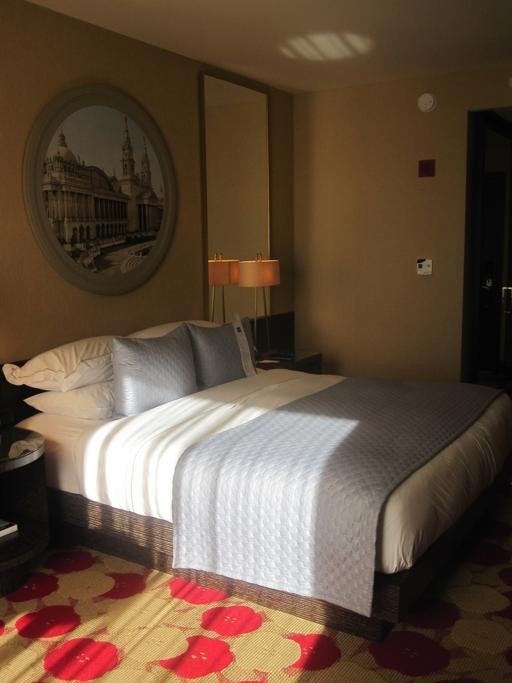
[21,83,178,296]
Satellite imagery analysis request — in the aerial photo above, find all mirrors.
[198,66,273,324]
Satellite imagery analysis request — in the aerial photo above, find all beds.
[0,360,512,640]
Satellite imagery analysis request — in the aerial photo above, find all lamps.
[239,253,281,358]
[208,252,240,324]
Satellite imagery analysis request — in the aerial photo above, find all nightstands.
[0,425,52,598]
[256,346,322,375]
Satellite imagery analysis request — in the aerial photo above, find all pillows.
[1,314,259,420]
